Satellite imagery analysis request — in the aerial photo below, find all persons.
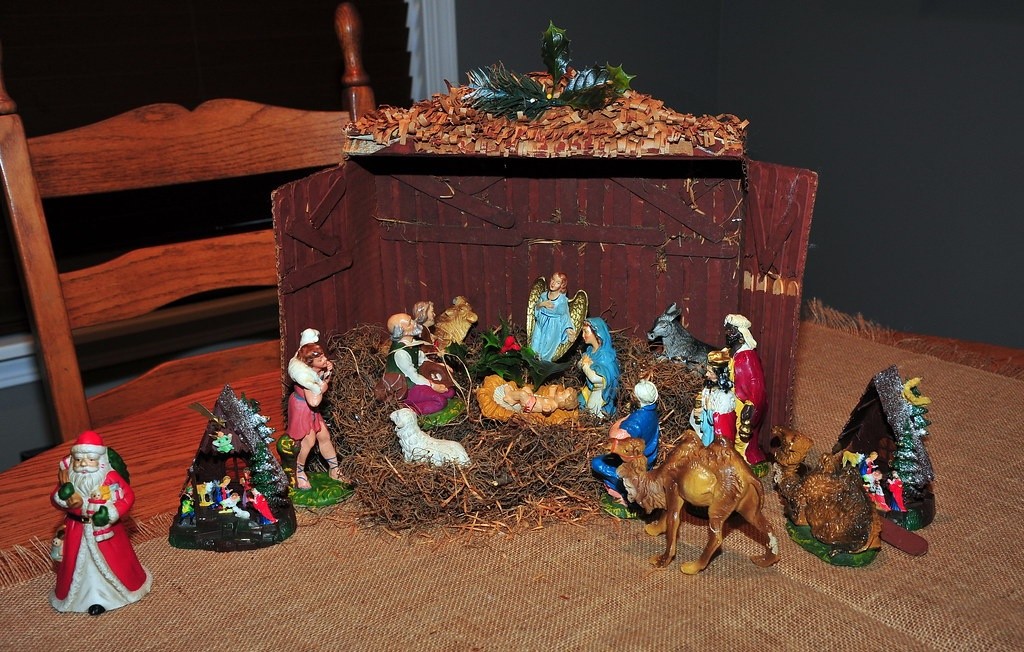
[286,343,351,491]
[180,468,276,528]
[503,385,579,416]
[690,348,738,450]
[859,452,907,513]
[579,316,620,417]
[724,314,767,466]
[530,271,578,363]
[591,379,659,507]
[52,430,152,613]
[386,301,456,415]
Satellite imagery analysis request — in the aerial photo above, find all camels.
[379,295,478,370]
[601,424,878,576]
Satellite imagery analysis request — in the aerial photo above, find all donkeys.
[646,301,721,367]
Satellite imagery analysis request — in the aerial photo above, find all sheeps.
[390,408,471,466]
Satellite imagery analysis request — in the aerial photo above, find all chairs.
[1,3,379,442]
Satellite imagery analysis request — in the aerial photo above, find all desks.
[0,317,1024,652]
